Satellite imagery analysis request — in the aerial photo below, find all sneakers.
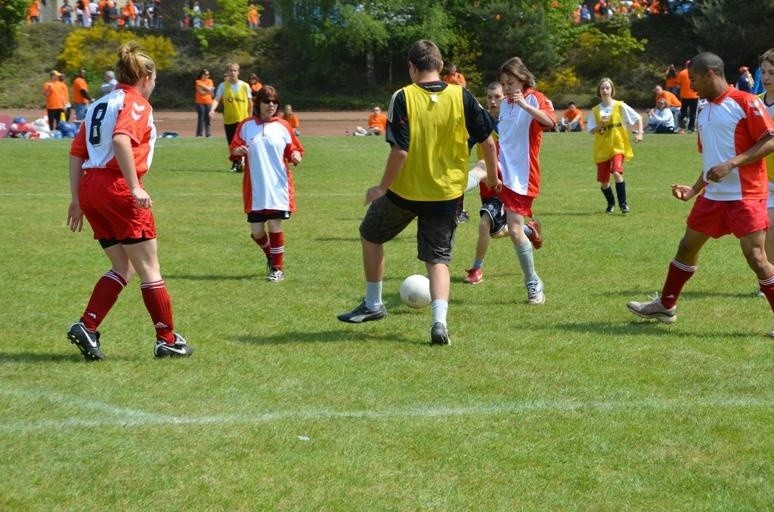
[607,204,614,213]
[337,301,386,323]
[620,203,630,213]
[154,333,193,359]
[229,163,242,172]
[68,323,105,362]
[527,282,545,304]
[464,268,482,284]
[431,323,450,345]
[527,219,542,248]
[628,291,675,322]
[267,269,283,282]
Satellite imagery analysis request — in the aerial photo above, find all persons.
[461,79,544,285]
[334,37,504,348]
[65,42,195,362]
[280,103,301,137]
[644,59,764,137]
[571,0,695,26]
[585,77,644,217]
[25,0,214,34]
[207,62,254,172]
[623,51,774,339]
[194,67,217,139]
[209,72,231,119]
[248,72,266,115]
[246,2,261,30]
[228,82,305,284]
[353,105,386,136]
[559,99,585,134]
[461,55,557,307]
[4,65,119,141]
[754,46,774,296]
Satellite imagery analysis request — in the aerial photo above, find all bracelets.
[638,130,644,135]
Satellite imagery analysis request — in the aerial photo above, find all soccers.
[400,274,433,308]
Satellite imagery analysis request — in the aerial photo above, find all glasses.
[261,98,279,105]
